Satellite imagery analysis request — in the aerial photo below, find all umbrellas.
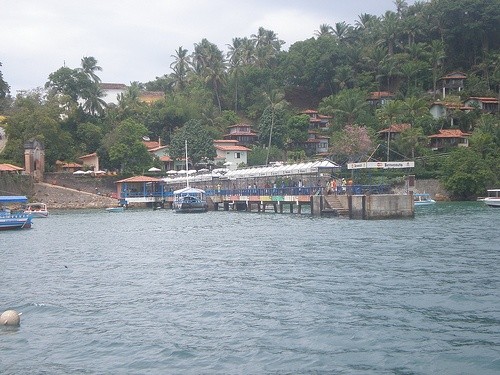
[74,169,106,175]
[148,167,161,171]
[166,169,196,174]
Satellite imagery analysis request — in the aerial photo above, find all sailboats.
[171,138,209,213]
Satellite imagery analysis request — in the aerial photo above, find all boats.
[483,188,500,207]
[0,195,33,231]
[411,193,437,206]
[22,202,49,218]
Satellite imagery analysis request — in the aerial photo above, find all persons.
[325,178,337,194]
[318,179,322,189]
[341,178,347,192]
[348,179,353,191]
[248,184,251,192]
[298,180,302,192]
[281,182,284,192]
[253,183,257,193]
[273,182,277,192]
